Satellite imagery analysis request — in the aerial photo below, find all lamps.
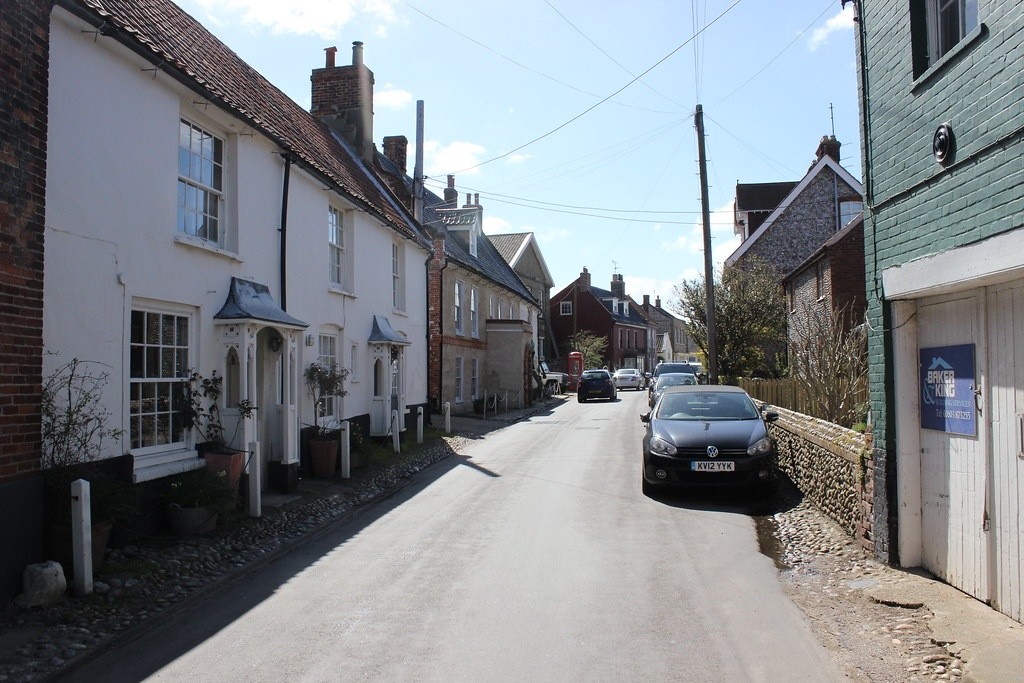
[270,338,281,352]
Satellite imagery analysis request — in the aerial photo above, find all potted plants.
[179,368,261,486]
[351,420,368,471]
[30,355,128,575]
[163,470,234,533]
[304,360,350,479]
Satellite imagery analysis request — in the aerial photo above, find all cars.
[641,383,781,493]
[539,358,710,409]
